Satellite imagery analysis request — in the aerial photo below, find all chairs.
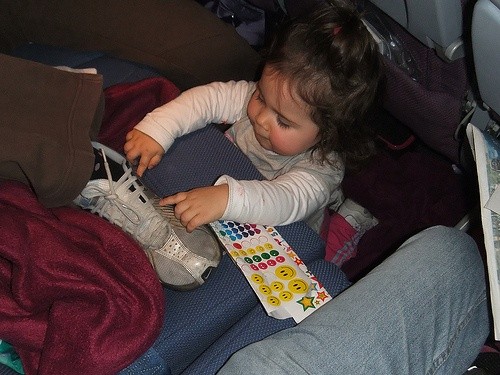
[0,0,500,375]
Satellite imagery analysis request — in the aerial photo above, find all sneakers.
[337,198,379,233]
[73,141,222,292]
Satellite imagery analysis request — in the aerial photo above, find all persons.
[215,225,490,375]
[123,5,383,236]
